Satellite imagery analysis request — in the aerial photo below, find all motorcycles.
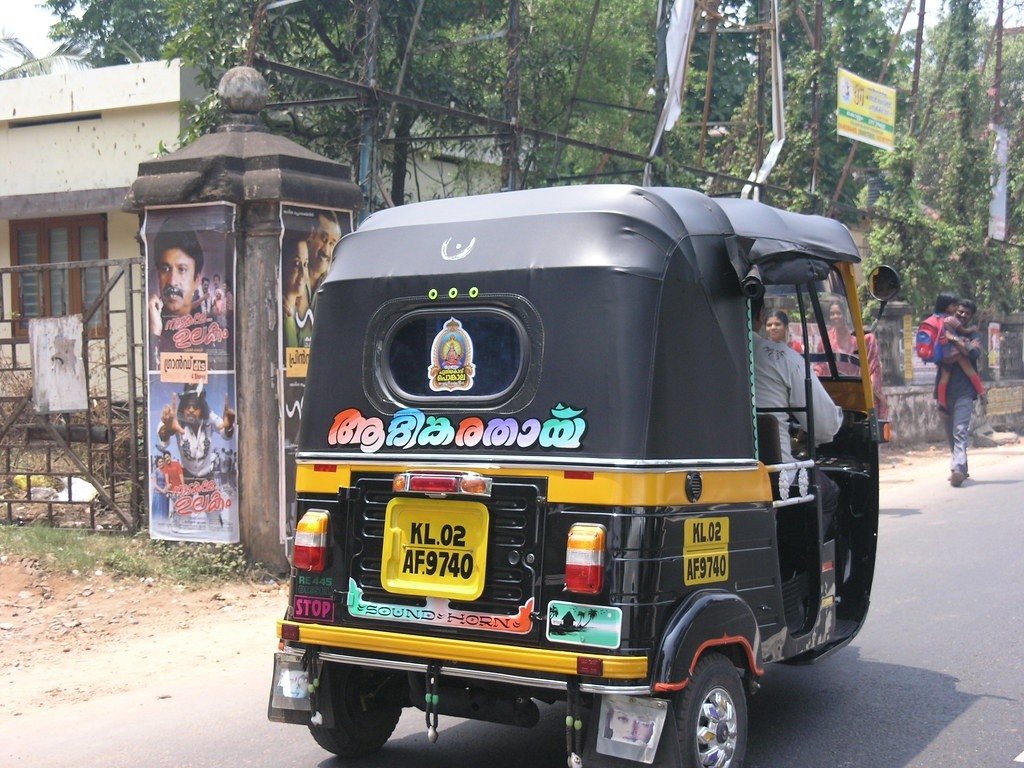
[264,184,900,768]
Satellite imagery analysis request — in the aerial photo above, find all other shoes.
[938,405,951,417]
[948,470,969,487]
[980,394,988,405]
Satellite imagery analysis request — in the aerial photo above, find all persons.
[609,710,653,745]
[212,447,238,491]
[764,311,803,352]
[149,215,232,370]
[153,451,184,521]
[283,209,342,350]
[752,288,843,538]
[814,299,881,416]
[157,383,237,524]
[934,294,986,409]
[933,300,981,486]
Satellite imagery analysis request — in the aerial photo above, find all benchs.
[758,416,816,498]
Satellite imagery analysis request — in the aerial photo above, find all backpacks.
[916,313,948,363]
